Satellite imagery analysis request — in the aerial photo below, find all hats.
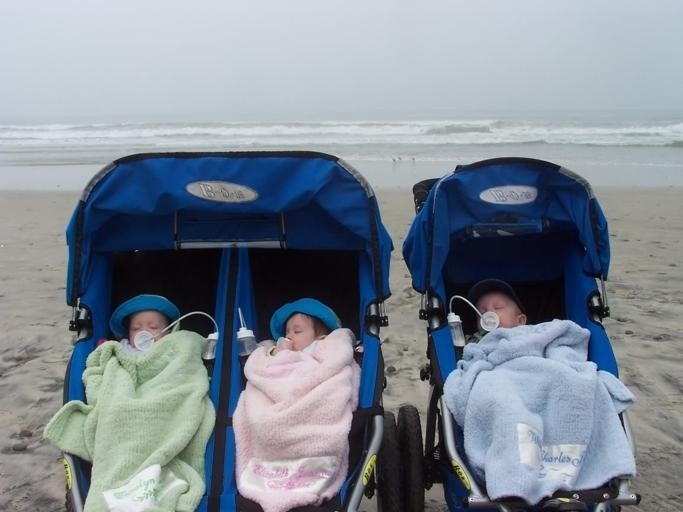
[466,277,528,317]
[108,292,184,339]
[268,295,343,341]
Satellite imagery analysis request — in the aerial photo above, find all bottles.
[447,313,466,347]
[236,327,256,356]
[202,331,218,360]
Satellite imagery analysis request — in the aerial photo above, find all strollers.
[398,156,642,511]
[63,152,401,512]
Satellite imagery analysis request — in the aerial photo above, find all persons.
[267,297,343,358]
[456,278,528,366]
[109,293,180,353]
